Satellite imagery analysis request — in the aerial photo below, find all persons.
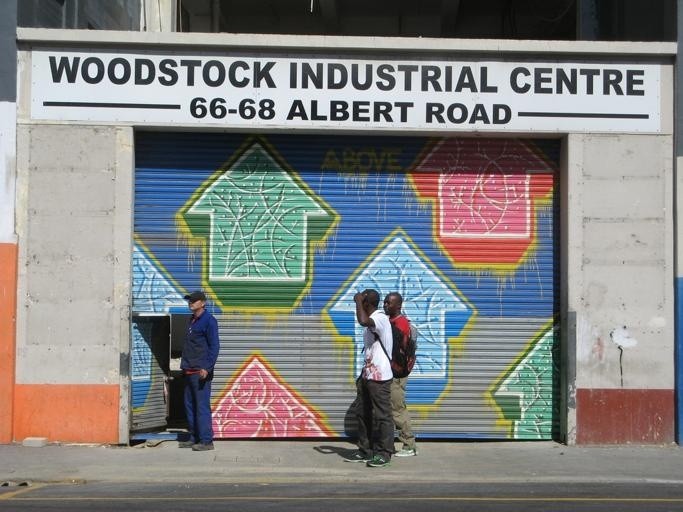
[382,292,420,457]
[344,288,396,467]
[178,290,220,451]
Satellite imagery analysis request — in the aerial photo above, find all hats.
[185,291,205,301]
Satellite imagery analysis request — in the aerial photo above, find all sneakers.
[395,449,418,456]
[345,452,371,463]
[179,439,213,451]
[368,454,388,466]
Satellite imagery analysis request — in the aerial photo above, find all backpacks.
[370,321,415,378]
